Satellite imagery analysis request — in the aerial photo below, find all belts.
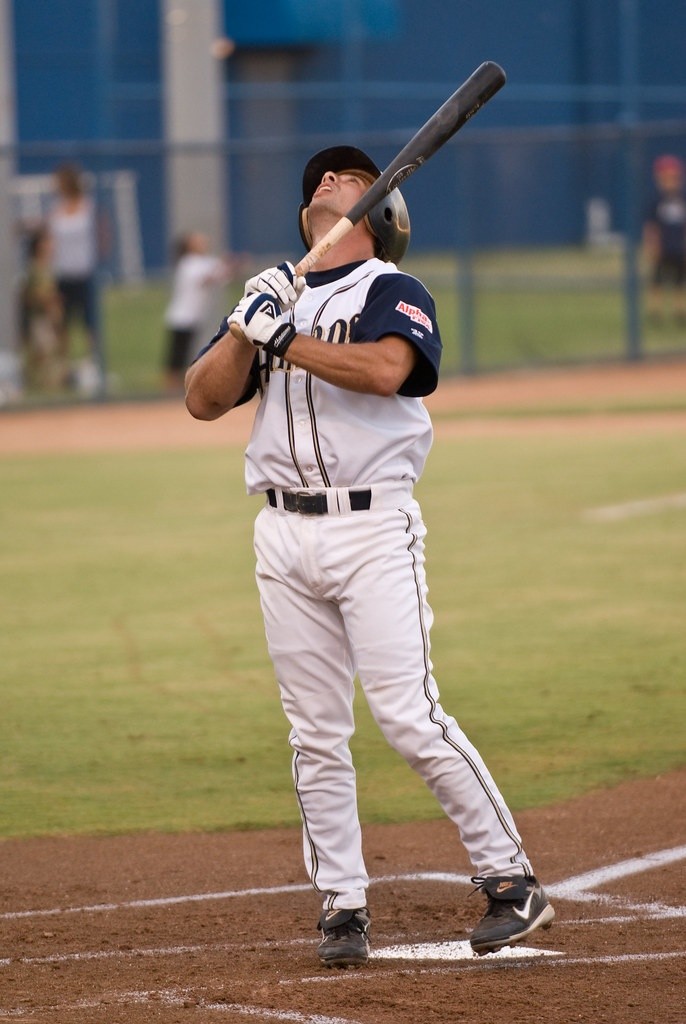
[267,488,370,515]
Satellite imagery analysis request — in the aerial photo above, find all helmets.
[299,145,409,267]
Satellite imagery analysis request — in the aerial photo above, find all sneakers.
[317,908,372,966]
[467,876,554,950]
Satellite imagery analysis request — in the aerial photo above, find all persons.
[641,155,686,316]
[184,144,555,967]
[15,166,226,402]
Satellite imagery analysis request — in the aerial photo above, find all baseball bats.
[296,60,506,277]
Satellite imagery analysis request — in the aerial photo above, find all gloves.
[244,262,305,315]
[226,292,297,356]
